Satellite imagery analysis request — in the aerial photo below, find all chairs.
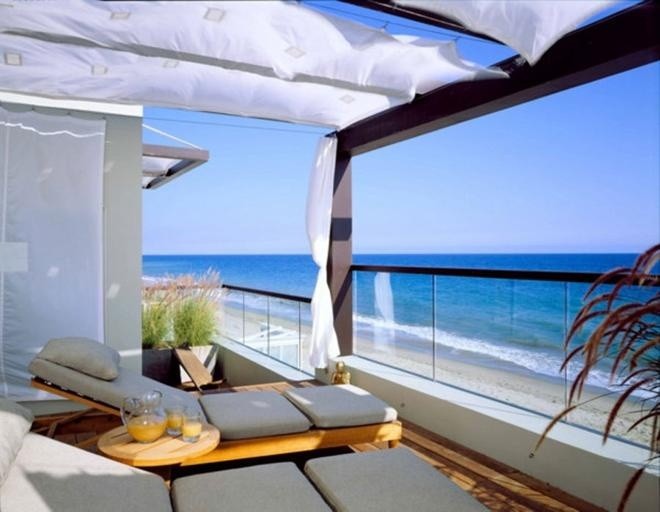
[0,432,492,512]
[30,357,402,467]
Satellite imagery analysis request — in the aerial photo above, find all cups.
[165,405,187,436]
[183,411,202,443]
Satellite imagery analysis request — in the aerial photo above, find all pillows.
[0,398,35,488]
[36,335,121,381]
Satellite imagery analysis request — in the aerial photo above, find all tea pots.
[119,389,166,443]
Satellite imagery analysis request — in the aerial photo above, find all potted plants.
[143,296,227,387]
